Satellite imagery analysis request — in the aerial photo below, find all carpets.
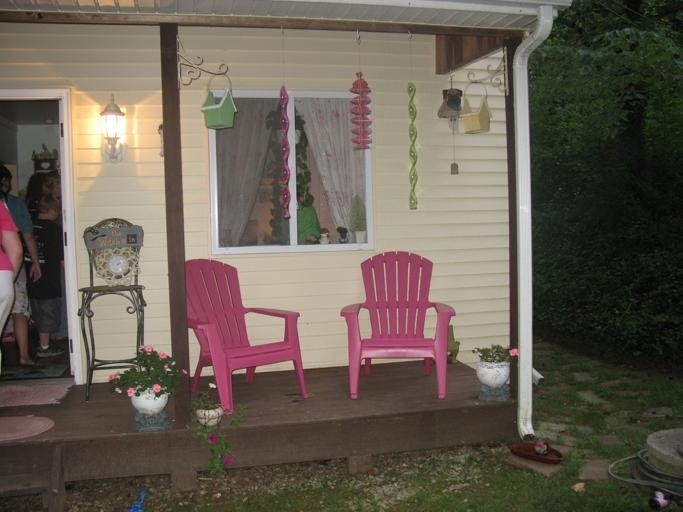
[0,415,55,443]
[0,378,75,408]
[0,350,70,380]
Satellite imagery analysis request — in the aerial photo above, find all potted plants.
[347,193,367,243]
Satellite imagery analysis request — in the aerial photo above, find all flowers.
[184,383,247,478]
[108,344,189,400]
[472,344,520,364]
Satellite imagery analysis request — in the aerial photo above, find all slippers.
[20,361,47,369]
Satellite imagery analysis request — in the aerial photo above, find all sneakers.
[37,344,65,358]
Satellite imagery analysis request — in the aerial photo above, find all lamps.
[99,93,128,163]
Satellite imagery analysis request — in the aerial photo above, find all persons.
[37,170,68,341]
[0,161,23,346]
[21,171,52,329]
[24,192,65,359]
[270,184,322,246]
[0,164,41,370]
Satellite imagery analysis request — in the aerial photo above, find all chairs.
[340,250,456,399]
[185,259,315,417]
[75,217,148,400]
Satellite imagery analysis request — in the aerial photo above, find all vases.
[474,359,512,403]
[195,406,224,427]
[130,389,172,431]
